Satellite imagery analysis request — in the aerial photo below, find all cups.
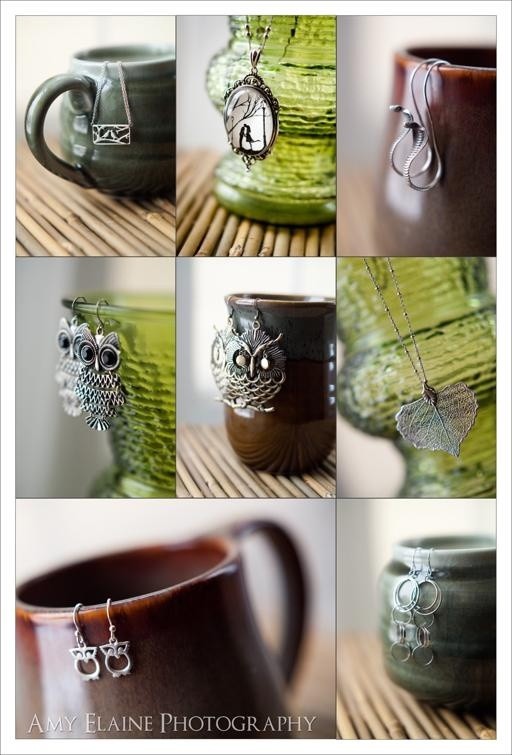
[16,517,309,739]
[336,256,496,498]
[23,42,176,199]
[60,289,176,498]
[376,533,496,703]
[374,46,497,256]
[204,15,336,226]
[223,292,335,475]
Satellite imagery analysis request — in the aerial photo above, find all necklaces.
[90,61,133,145]
[362,257,480,458]
[223,15,280,172]
